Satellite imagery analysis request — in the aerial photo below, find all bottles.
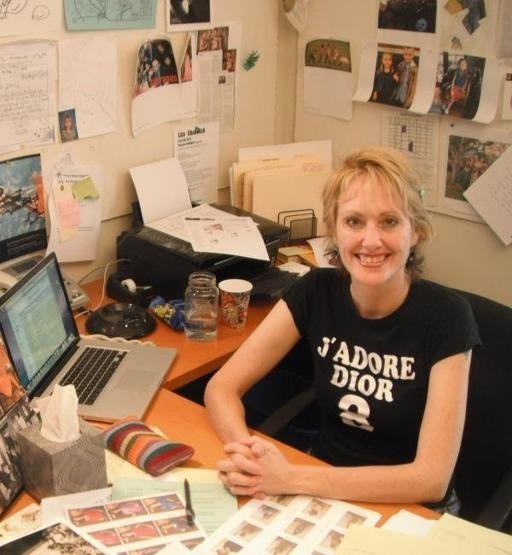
[183,271,218,341]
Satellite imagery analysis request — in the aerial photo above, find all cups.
[219,277,253,330]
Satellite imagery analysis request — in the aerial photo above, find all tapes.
[121,279,151,293]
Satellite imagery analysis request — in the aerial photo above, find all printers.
[116,187,301,301]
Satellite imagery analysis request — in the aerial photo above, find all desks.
[0,385,511,555]
[71,265,292,390]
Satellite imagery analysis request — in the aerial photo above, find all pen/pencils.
[184,478,194,526]
[186,217,215,221]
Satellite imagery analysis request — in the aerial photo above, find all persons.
[0,412,27,506]
[307,44,340,65]
[202,148,483,517]
[368,52,400,104]
[138,44,176,88]
[199,31,208,51]
[441,58,470,115]
[71,497,202,555]
[396,47,418,109]
[226,50,235,72]
[210,28,221,50]
[61,114,78,143]
[217,495,364,555]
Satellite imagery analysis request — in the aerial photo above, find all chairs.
[255,278,512,534]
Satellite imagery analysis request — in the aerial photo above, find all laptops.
[0,252,177,424]
